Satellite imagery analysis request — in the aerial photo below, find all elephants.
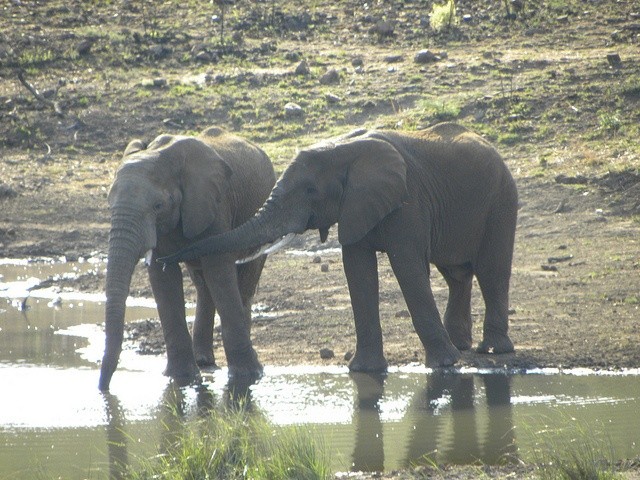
[156,121,519,372]
[99,126,277,392]
